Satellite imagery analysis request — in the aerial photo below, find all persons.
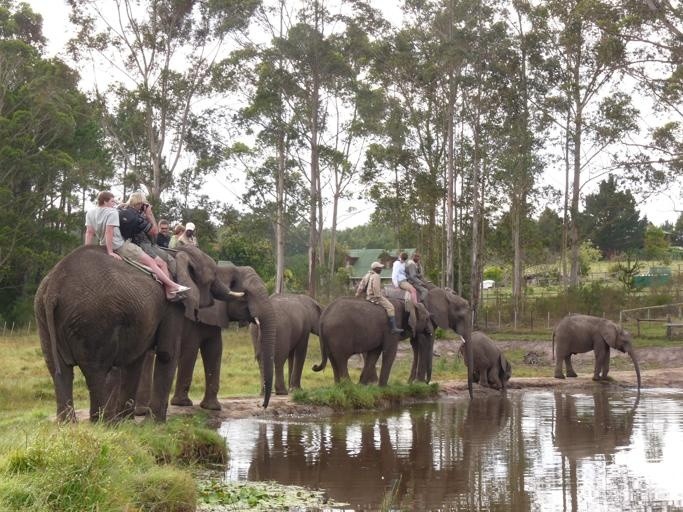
[355,262,404,335]
[82,191,199,303]
[404,253,428,308]
[391,253,422,308]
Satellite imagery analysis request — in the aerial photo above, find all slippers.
[117,205,145,239]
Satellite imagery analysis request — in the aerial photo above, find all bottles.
[186,222,196,232]
[129,192,146,205]
[371,261,385,270]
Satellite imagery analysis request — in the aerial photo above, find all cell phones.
[141,204,149,211]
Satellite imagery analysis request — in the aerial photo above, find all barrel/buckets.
[167,292,190,303]
[168,286,192,294]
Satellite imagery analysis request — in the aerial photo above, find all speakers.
[388,315,405,335]
